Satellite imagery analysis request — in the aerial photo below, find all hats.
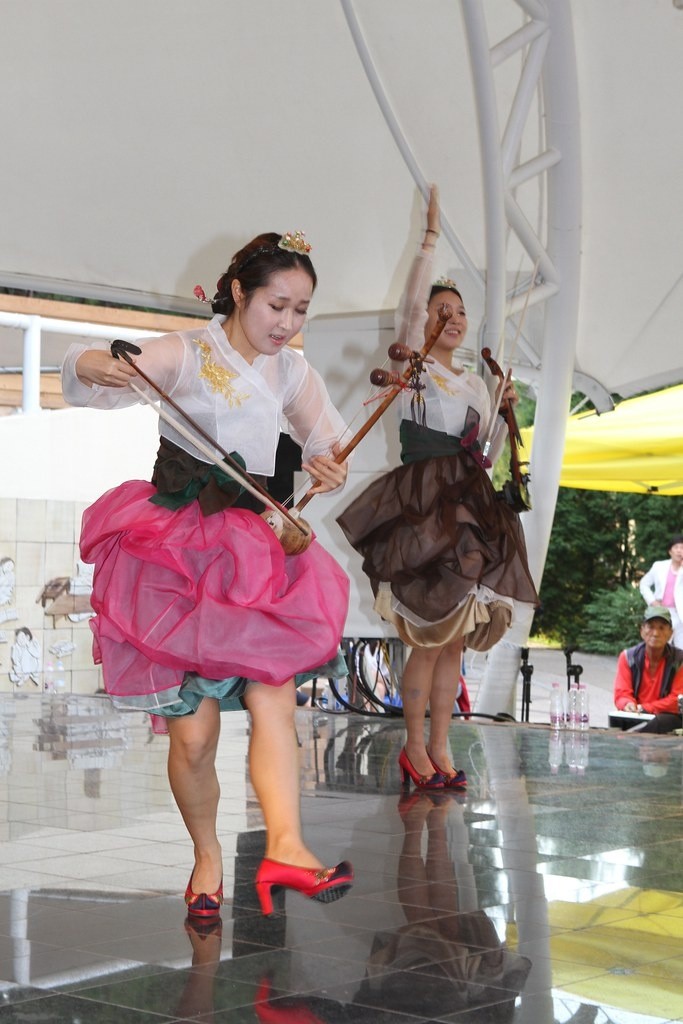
[642,605,672,628]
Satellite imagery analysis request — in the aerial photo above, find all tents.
[516,382,683,498]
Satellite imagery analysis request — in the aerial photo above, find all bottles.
[566,683,578,730]
[576,732,588,775]
[566,731,577,772]
[550,681,566,730]
[549,731,563,775]
[575,684,589,729]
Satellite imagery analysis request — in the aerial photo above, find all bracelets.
[422,244,436,249]
[427,228,441,238]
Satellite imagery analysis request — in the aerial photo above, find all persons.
[613,606,683,735]
[639,537,683,649]
[59,231,356,919]
[339,182,522,792]
[355,793,531,1022]
[292,638,477,725]
[177,918,353,1024]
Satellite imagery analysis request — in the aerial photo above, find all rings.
[513,390,517,395]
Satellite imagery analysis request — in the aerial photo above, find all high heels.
[397,783,468,822]
[184,863,224,917]
[253,970,350,1024]
[183,916,222,949]
[424,745,467,787]
[398,746,445,788]
[253,857,354,915]
